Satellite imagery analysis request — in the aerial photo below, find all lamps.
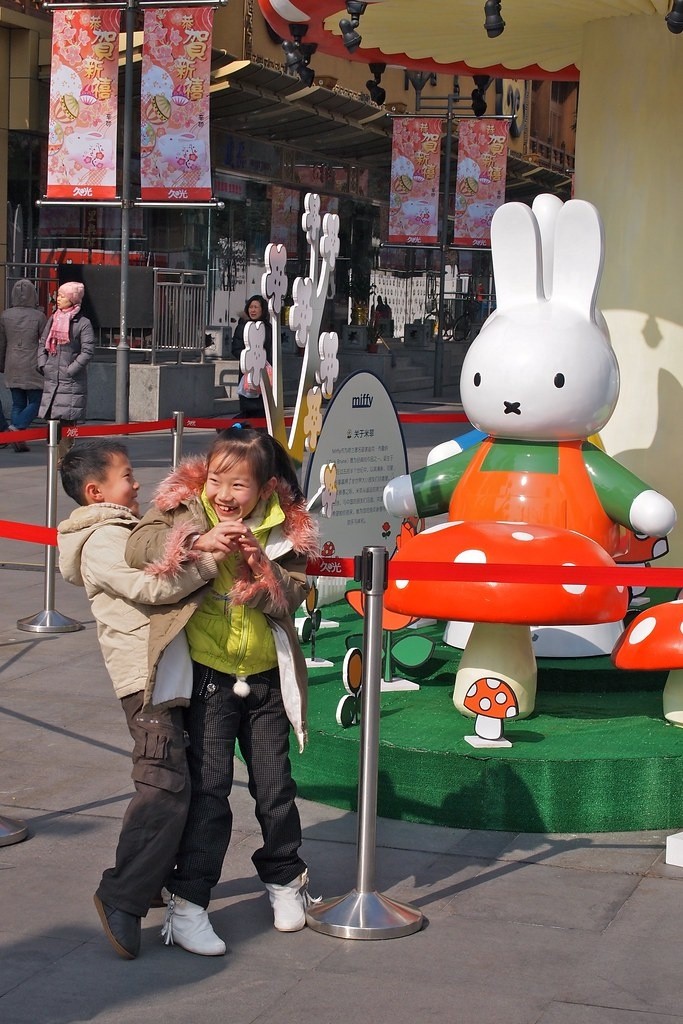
[481,1,506,38]
[295,41,318,88]
[281,24,308,66]
[338,0,367,54]
[363,61,387,105]
[470,74,493,119]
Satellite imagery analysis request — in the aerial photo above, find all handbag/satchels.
[246,361,272,393]
[237,375,260,400]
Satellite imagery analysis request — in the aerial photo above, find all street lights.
[403,67,493,397]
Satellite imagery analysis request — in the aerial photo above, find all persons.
[229,295,274,421]
[52,439,246,959]
[35,281,96,473]
[123,421,326,957]
[0,279,47,454]
[0,400,12,450]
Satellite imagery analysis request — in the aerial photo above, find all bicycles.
[451,290,487,342]
[422,295,453,343]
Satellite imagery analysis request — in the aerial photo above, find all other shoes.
[12,438,31,452]
[94,895,141,959]
[265,868,310,933]
[163,898,227,955]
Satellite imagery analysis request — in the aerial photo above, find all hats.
[57,281,84,305]
